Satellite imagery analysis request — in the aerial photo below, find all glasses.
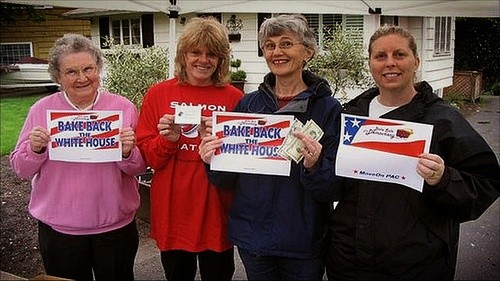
[262,40,308,52]
[57,63,97,79]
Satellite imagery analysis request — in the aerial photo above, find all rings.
[431,170,435,177]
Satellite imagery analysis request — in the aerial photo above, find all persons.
[10,34,147,281]
[136,16,244,281]
[324,26,500,281]
[199,16,345,281]
